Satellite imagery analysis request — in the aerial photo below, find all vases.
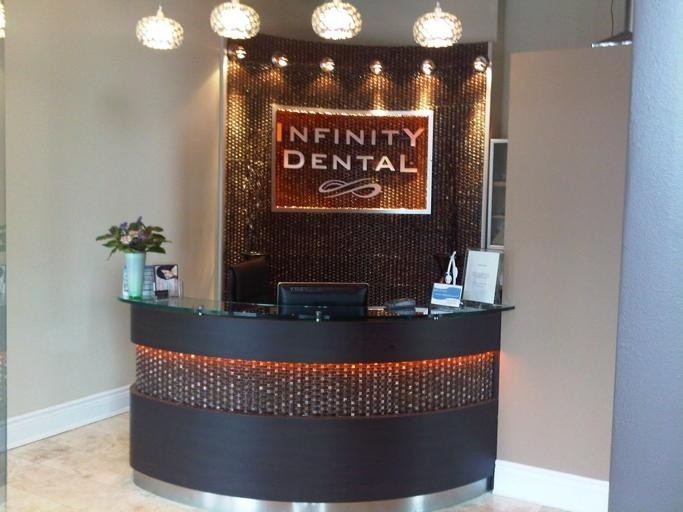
[125,253,145,299]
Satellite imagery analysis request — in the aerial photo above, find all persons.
[157,265,178,280]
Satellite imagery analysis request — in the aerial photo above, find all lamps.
[209,0,261,40]
[413,0,463,48]
[592,0,634,48]
[311,0,363,40]
[136,0,184,50]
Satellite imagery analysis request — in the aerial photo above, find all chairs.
[229,256,274,304]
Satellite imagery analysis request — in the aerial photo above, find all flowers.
[96,216,173,261]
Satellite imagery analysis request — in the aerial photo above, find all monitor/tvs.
[277,281,369,307]
[461,247,504,307]
